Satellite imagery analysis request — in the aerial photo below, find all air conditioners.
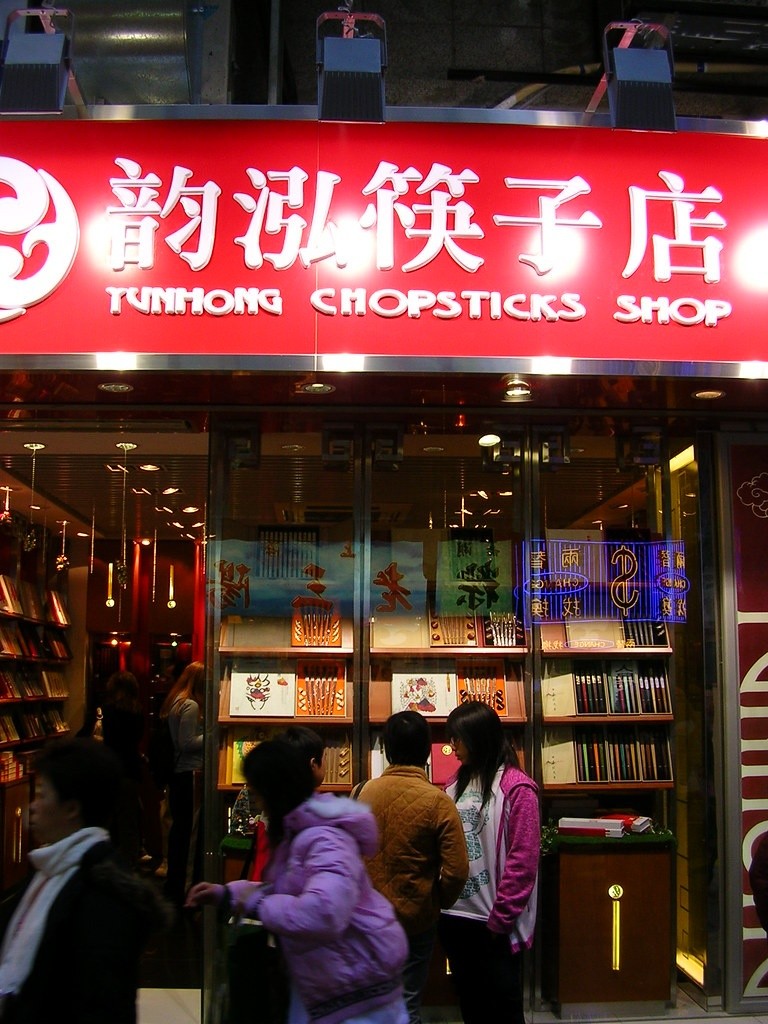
[273,500,414,526]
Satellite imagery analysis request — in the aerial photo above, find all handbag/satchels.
[204,885,269,1024]
[90,707,106,758]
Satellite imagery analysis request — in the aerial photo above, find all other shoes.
[152,857,168,878]
[137,854,153,863]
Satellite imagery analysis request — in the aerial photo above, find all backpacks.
[148,698,183,775]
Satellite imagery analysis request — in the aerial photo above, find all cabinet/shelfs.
[0,516,678,1020]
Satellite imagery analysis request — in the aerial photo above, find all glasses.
[450,736,461,747]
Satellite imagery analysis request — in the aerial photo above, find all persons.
[0,733,172,1024]
[441,700,540,1024]
[86,673,146,869]
[187,739,409,1024]
[348,710,469,1024]
[159,662,206,902]
[207,723,325,1023]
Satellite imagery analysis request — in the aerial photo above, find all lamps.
[602,17,678,132]
[314,12,389,125]
[0,7,76,115]
